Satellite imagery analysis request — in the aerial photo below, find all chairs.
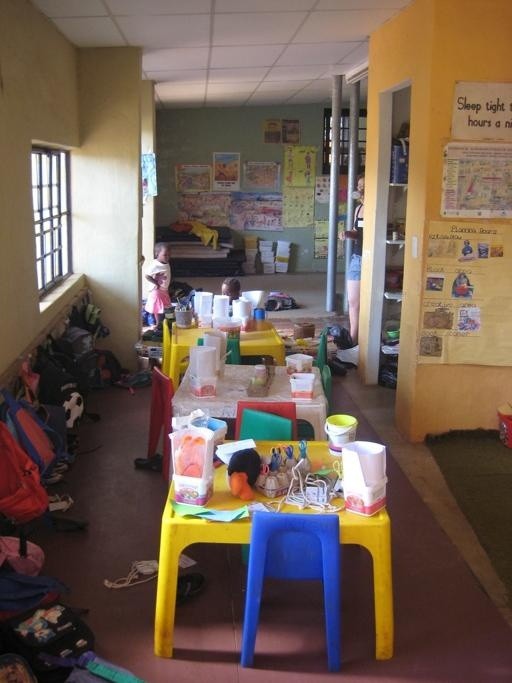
[301,365,332,437]
[148,366,175,482]
[234,400,298,440]
[240,510,343,671]
[197,339,241,365]
[239,408,293,440]
[312,334,327,374]
[162,319,189,378]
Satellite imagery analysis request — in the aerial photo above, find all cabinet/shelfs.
[380,183,406,354]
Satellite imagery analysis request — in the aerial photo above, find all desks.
[170,359,328,441]
[170,321,285,388]
[153,440,393,663]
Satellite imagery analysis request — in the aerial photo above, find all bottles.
[255,365,267,386]
[256,456,311,499]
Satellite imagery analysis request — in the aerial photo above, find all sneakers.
[176,571,207,600]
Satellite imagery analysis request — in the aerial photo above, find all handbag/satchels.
[392,139,409,183]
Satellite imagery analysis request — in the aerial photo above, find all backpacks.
[265,291,296,309]
[102,348,151,388]
[1,303,100,682]
[170,279,198,302]
[64,650,143,683]
[328,325,355,372]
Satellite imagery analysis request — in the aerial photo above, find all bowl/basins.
[387,328,400,339]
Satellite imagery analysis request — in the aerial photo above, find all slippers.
[134,454,161,470]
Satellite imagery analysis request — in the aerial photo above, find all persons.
[221,278,240,305]
[145,241,172,330]
[338,174,365,347]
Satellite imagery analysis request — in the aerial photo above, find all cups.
[254,308,265,319]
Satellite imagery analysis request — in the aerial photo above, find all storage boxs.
[244,235,291,275]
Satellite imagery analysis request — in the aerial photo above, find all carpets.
[1,318,512,683]
[423,430,512,601]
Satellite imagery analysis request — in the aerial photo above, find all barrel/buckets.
[175,310,193,328]
[497,411,512,448]
[324,414,358,452]
[340,441,388,517]
